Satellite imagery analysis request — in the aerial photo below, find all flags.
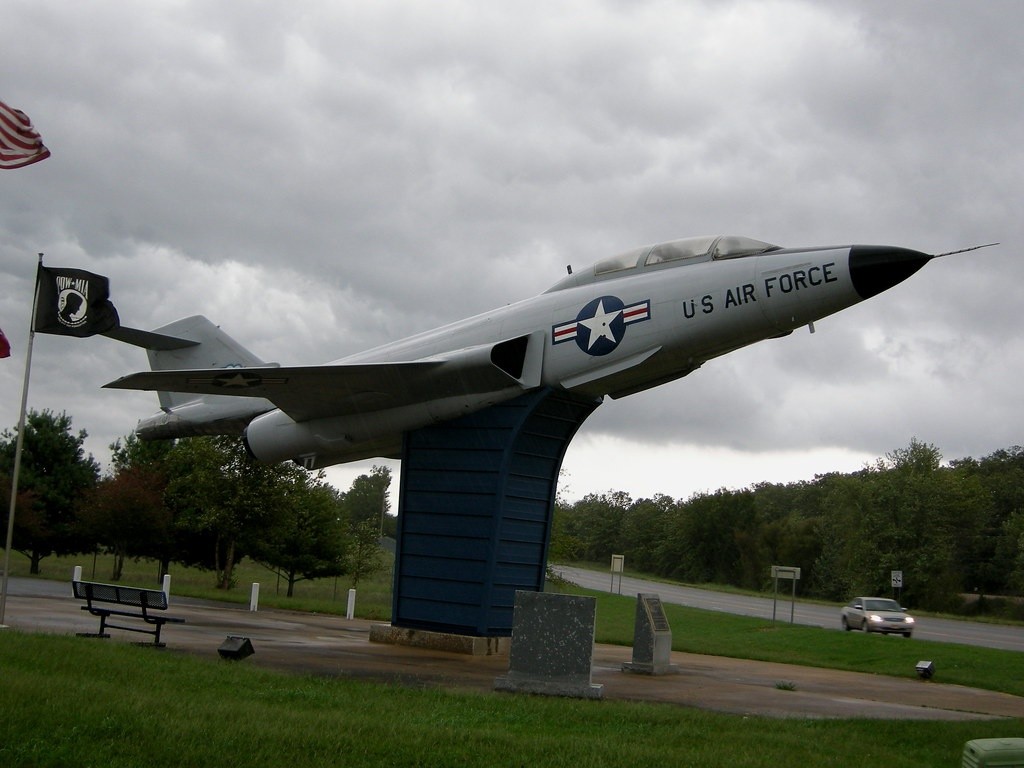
[0,329,11,359]
[0,101,55,171]
[30,260,122,339]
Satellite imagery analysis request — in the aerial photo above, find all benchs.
[73,581,185,648]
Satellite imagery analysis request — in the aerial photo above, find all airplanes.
[95,235,1000,638]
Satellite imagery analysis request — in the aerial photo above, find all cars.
[840,597,916,638]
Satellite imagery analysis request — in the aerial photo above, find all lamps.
[916,661,935,679]
[217,635,255,660]
